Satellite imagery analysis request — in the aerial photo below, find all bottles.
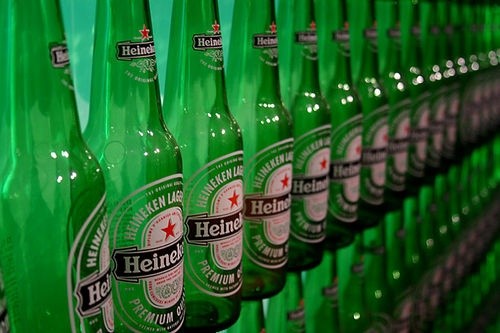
[0,0,500,333]
